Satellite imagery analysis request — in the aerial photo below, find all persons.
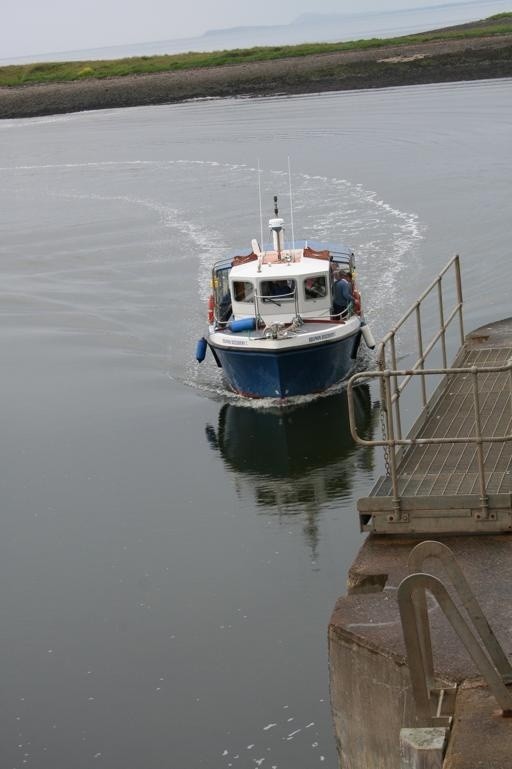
[213,263,362,332]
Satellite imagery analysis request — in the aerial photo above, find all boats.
[200,384,377,573]
[194,194,378,400]
